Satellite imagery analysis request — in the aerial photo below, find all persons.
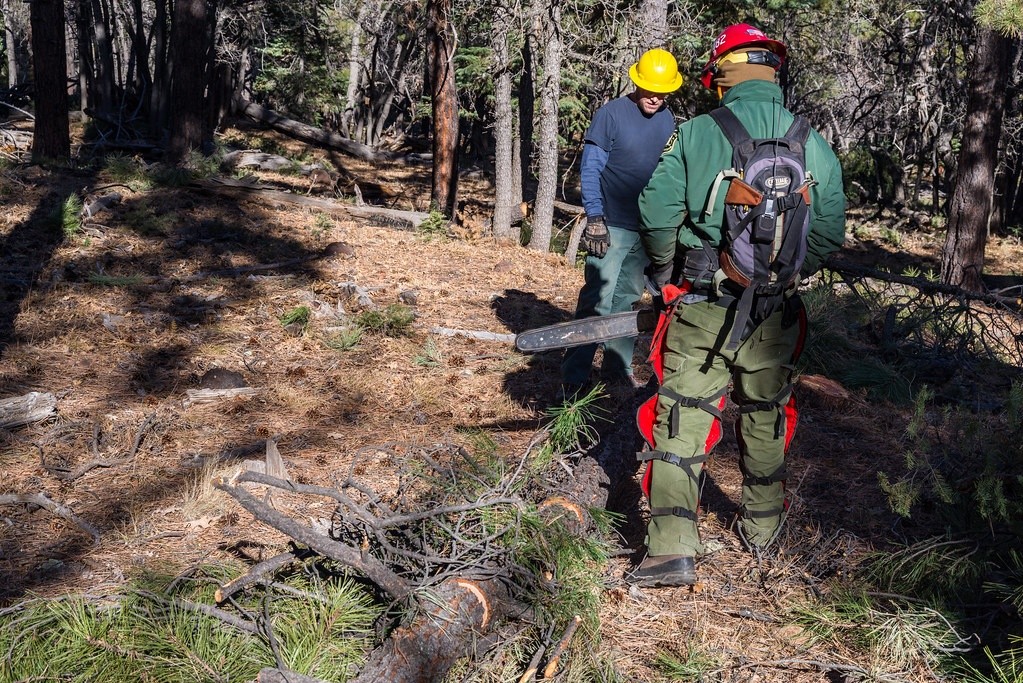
[621,24,848,587]
[561,49,683,399]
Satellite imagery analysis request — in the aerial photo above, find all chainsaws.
[515,302,659,353]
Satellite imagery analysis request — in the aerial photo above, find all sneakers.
[626,553,695,588]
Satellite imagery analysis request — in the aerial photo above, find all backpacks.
[707,106,812,296]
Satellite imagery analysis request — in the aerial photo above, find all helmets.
[699,24,787,92]
[629,48,683,93]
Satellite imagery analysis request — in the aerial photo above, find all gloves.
[585,216,612,259]
